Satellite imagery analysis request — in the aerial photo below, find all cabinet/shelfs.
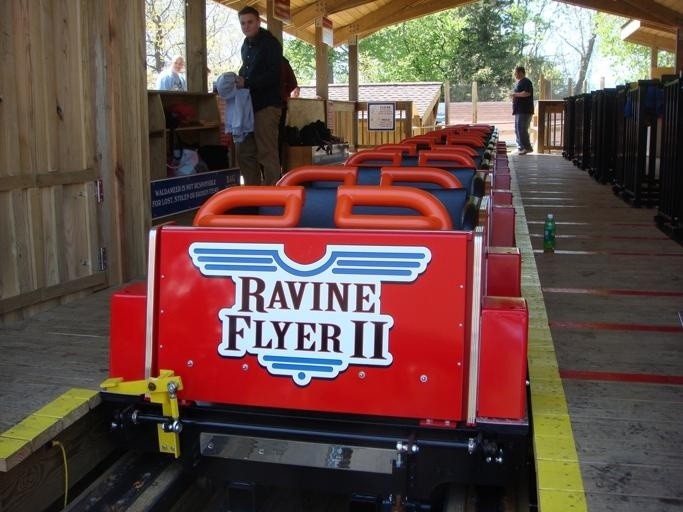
[146,91,240,221]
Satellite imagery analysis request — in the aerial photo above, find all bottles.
[543,213,555,249]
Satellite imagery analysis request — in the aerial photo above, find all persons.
[507,66,535,156]
[156,54,187,91]
[278,55,298,177]
[214,4,282,186]
[288,85,301,98]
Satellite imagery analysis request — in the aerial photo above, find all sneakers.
[519,149,533,155]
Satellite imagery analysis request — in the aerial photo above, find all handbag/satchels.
[161,102,231,178]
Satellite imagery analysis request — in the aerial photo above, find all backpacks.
[286,118,341,147]
